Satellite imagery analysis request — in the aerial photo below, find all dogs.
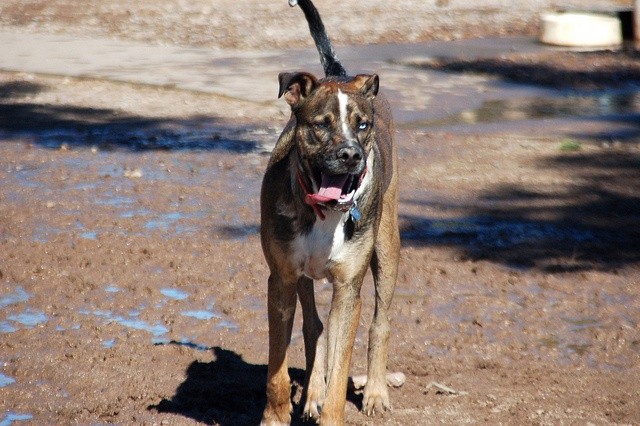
[260,0,401,426]
